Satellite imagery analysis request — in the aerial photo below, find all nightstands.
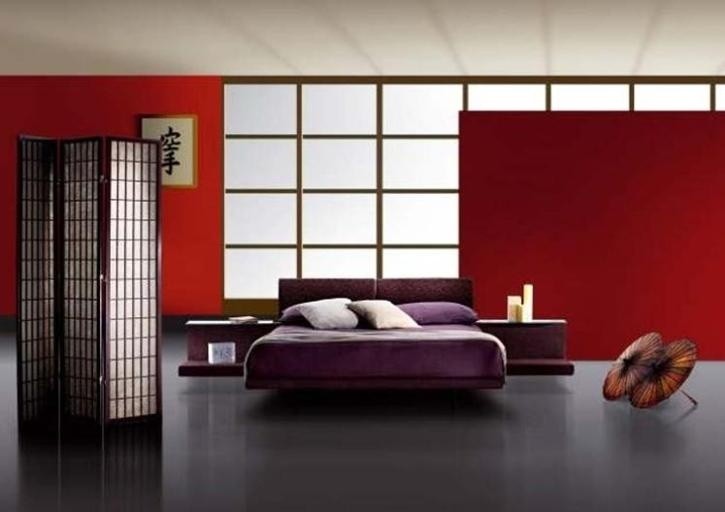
[178,319,279,377]
[475,319,574,375]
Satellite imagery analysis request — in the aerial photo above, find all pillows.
[293,297,362,331]
[279,306,307,323]
[346,299,423,331]
[396,302,479,325]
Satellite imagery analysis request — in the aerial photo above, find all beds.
[243,277,509,393]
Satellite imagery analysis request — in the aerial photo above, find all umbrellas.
[602,331,662,401]
[629,337,698,408]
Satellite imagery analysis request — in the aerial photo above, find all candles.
[523,284,534,321]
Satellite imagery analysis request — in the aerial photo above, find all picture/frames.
[136,112,199,190]
